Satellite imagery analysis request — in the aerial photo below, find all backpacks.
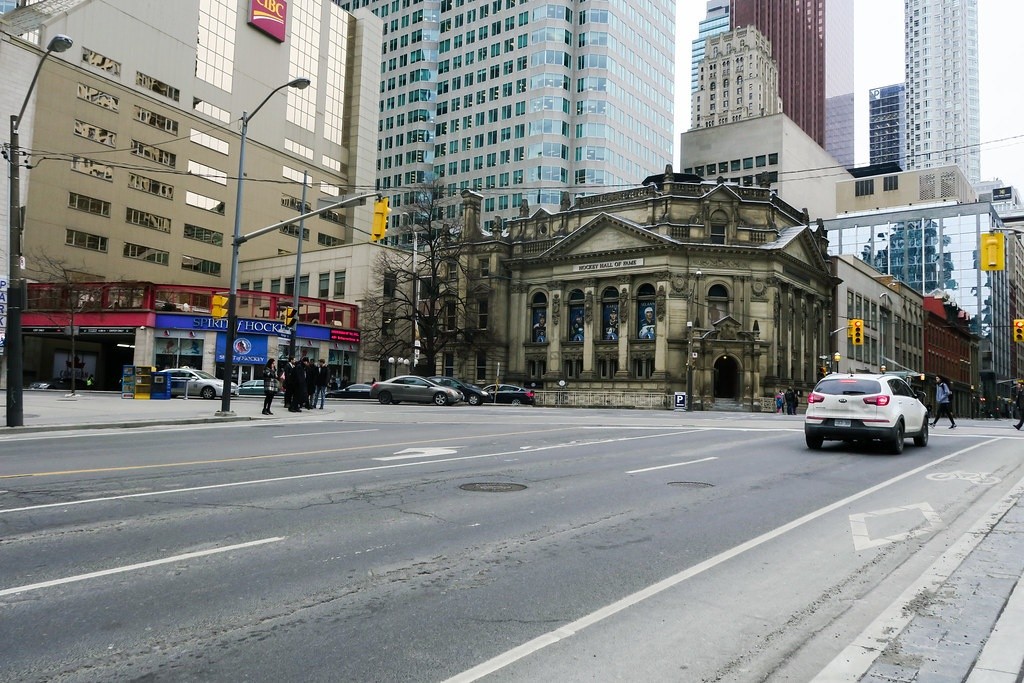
[942,381,952,401]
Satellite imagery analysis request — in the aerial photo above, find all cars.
[325,374,537,406]
[157,368,239,399]
[238,380,279,395]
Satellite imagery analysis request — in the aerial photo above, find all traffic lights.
[371,197,393,242]
[1013,319,1024,342]
[852,319,864,345]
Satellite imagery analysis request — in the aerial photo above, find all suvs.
[804,371,929,454]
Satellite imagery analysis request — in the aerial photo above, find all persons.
[604,308,618,339]
[533,315,547,338]
[928,375,957,429]
[639,307,655,339]
[262,359,279,415]
[776,388,799,415]
[571,314,584,340]
[283,356,331,412]
[1013,382,1024,430]
[331,378,338,390]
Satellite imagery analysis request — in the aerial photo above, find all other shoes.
[267,408,273,415]
[281,399,328,413]
[927,422,936,429]
[262,410,268,415]
[1013,425,1020,430]
[949,424,957,429]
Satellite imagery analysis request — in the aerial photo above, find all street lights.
[401,211,417,373]
[4,35,75,426]
[215,78,312,419]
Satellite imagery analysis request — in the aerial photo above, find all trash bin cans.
[151,372,171,400]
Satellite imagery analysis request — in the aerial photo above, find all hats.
[302,357,310,362]
[936,375,943,380]
[318,358,326,363]
